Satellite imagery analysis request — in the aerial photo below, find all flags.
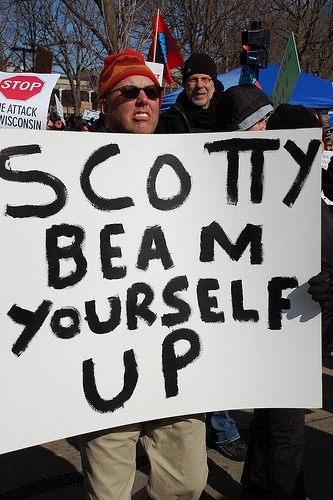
[148,12,184,87]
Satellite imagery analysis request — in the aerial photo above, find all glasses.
[187,78,212,84]
[106,85,164,100]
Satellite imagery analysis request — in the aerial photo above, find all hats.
[98,50,162,108]
[51,111,60,120]
[182,53,217,89]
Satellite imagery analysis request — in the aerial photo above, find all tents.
[159,62,333,121]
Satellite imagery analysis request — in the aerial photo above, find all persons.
[0,51,333,500]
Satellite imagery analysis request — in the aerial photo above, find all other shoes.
[215,438,248,461]
